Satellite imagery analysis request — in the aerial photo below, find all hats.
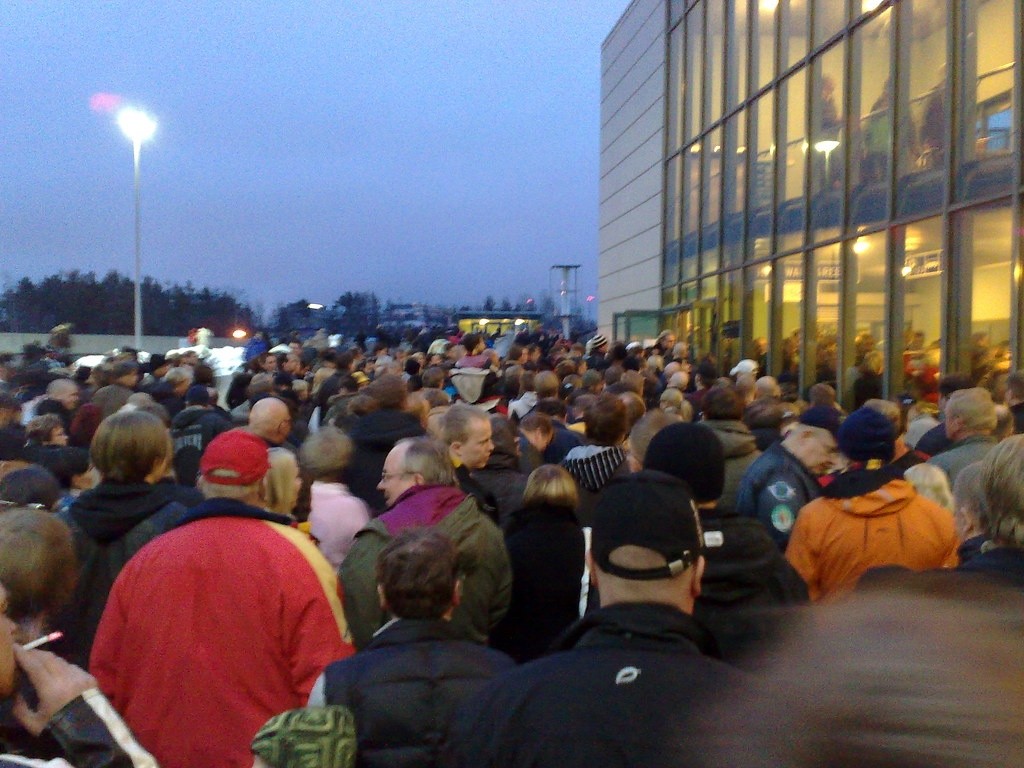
[249,704,357,768]
[147,354,171,372]
[730,360,759,377]
[798,404,848,435]
[591,469,701,581]
[201,430,270,485]
[838,409,895,463]
[351,371,370,384]
[642,422,725,504]
[590,334,606,349]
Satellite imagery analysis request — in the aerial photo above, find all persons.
[818,74,840,192]
[88,429,357,768]
[0,322,1024,669]
[432,470,764,768]
[0,509,158,767]
[251,532,521,768]
[865,77,920,179]
[923,77,947,165]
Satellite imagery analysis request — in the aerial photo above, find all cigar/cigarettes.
[22,631,63,651]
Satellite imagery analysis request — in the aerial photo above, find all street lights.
[117,106,160,350]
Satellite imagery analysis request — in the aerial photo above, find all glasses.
[378,470,417,482]
